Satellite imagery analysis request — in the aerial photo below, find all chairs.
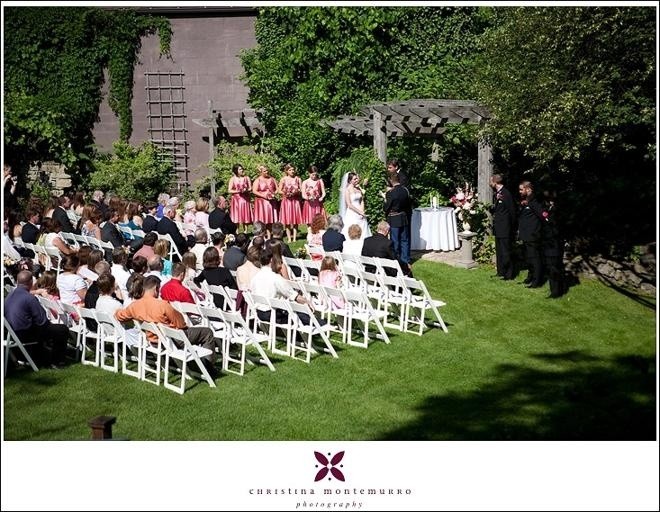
[4,225,448,394]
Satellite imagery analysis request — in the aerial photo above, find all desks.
[410,207,460,252]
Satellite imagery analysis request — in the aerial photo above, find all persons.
[4,159,423,376]
[540,190,569,299]
[517,180,542,288]
[489,174,517,281]
